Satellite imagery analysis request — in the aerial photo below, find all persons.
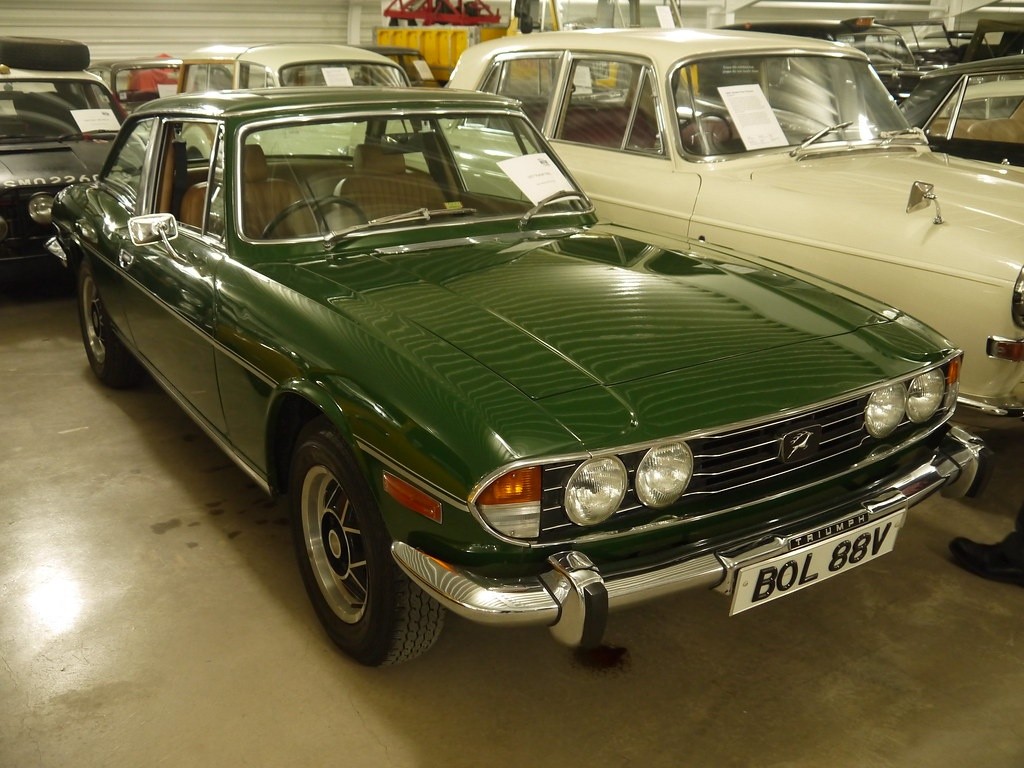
[949,498,1024,588]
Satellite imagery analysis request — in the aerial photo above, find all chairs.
[331,143,447,231]
[180,144,320,246]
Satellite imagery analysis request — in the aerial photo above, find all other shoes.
[949,537,1024,587]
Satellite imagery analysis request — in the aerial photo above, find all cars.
[0,34,150,285]
[404,28,1024,438]
[705,1,1024,169]
[55,88,989,673]
[356,45,444,91]
[178,39,412,160]
[87,57,236,120]
